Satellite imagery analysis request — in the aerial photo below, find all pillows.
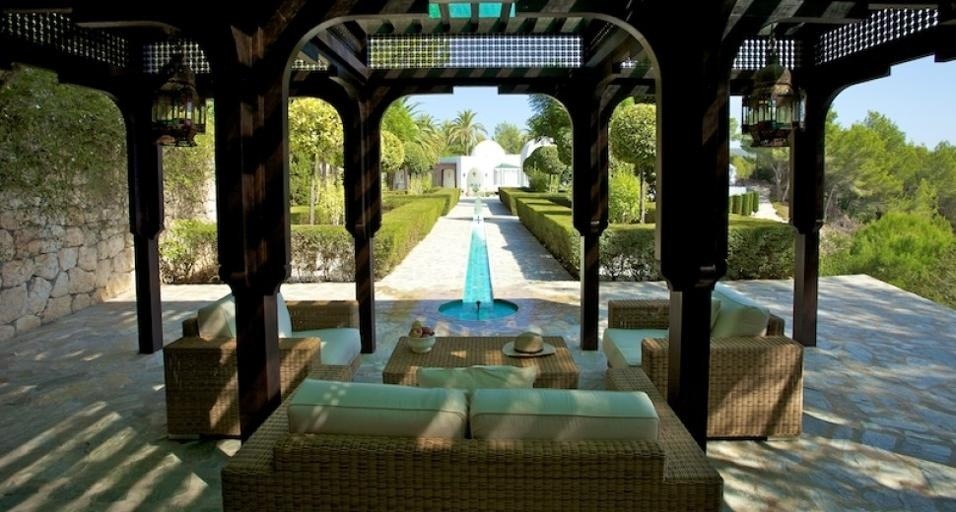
[667,296,722,338]
[416,366,538,388]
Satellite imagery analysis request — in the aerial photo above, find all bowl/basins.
[407,330,436,353]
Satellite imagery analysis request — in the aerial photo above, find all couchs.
[221,364,725,508]
[166,290,362,440]
[603,287,803,440]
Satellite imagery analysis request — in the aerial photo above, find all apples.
[409,320,433,337]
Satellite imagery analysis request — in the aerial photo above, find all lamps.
[740,23,808,148]
[148,37,208,149]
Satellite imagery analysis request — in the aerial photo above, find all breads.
[514,332,544,353]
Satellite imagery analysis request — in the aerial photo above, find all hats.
[502,332,555,358]
